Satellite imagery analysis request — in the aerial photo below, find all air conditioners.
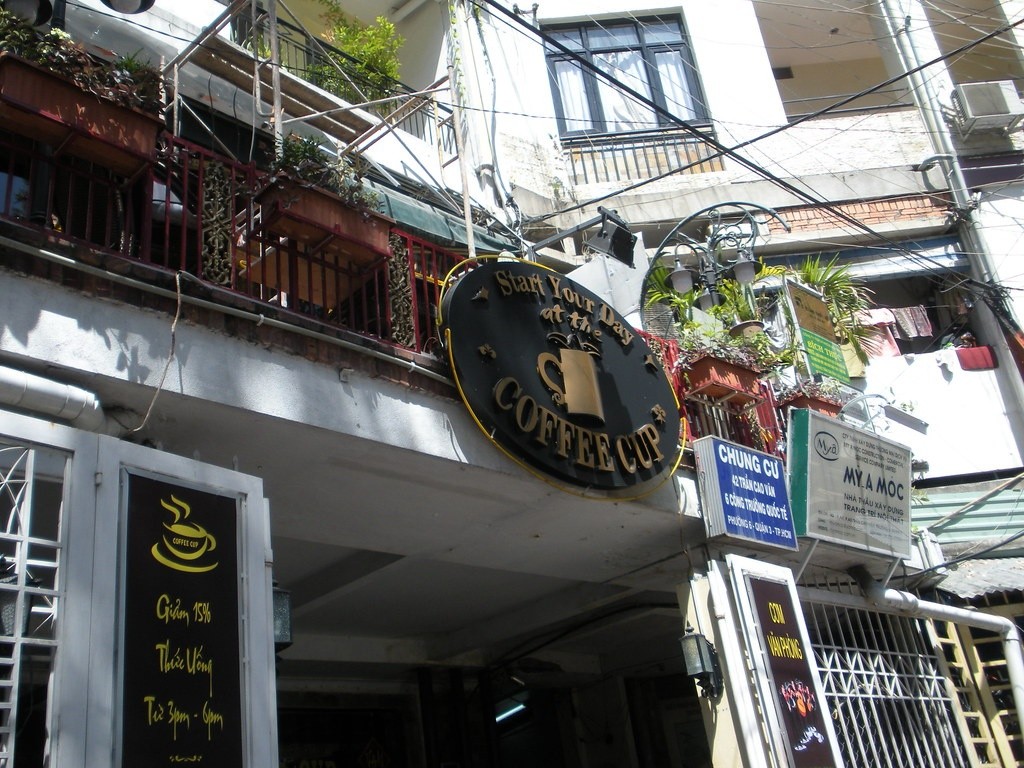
[949,79,1024,131]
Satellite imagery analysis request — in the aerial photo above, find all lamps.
[638,200,791,332]
[273,571,293,673]
[679,620,724,700]
[531,205,638,269]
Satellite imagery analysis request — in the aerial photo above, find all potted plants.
[0,9,164,177]
[706,280,767,358]
[776,379,843,419]
[254,131,397,271]
[674,318,803,407]
